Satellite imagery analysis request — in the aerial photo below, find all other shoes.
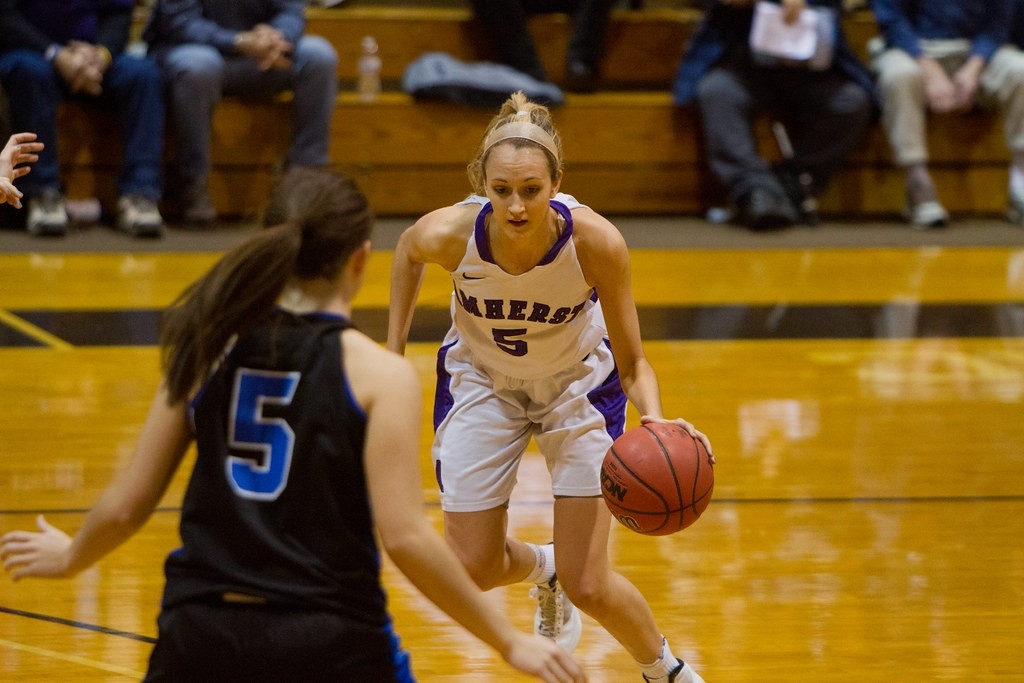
[184,196,216,225]
[117,194,163,237]
[910,200,949,229]
[27,194,67,235]
[747,188,793,226]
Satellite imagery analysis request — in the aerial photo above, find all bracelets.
[234,32,242,47]
[45,44,65,61]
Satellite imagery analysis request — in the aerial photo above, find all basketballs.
[599,423,715,536]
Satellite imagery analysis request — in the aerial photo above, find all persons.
[385,91,716,683]
[0,0,338,238]
[0,168,590,683]
[467,0,1024,232]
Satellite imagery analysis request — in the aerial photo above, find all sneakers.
[529,541,581,656]
[642,658,706,683]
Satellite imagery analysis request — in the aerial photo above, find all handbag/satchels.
[404,52,564,108]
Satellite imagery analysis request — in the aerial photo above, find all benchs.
[51,0,1024,225]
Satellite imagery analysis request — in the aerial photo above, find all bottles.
[356,36,382,94]
